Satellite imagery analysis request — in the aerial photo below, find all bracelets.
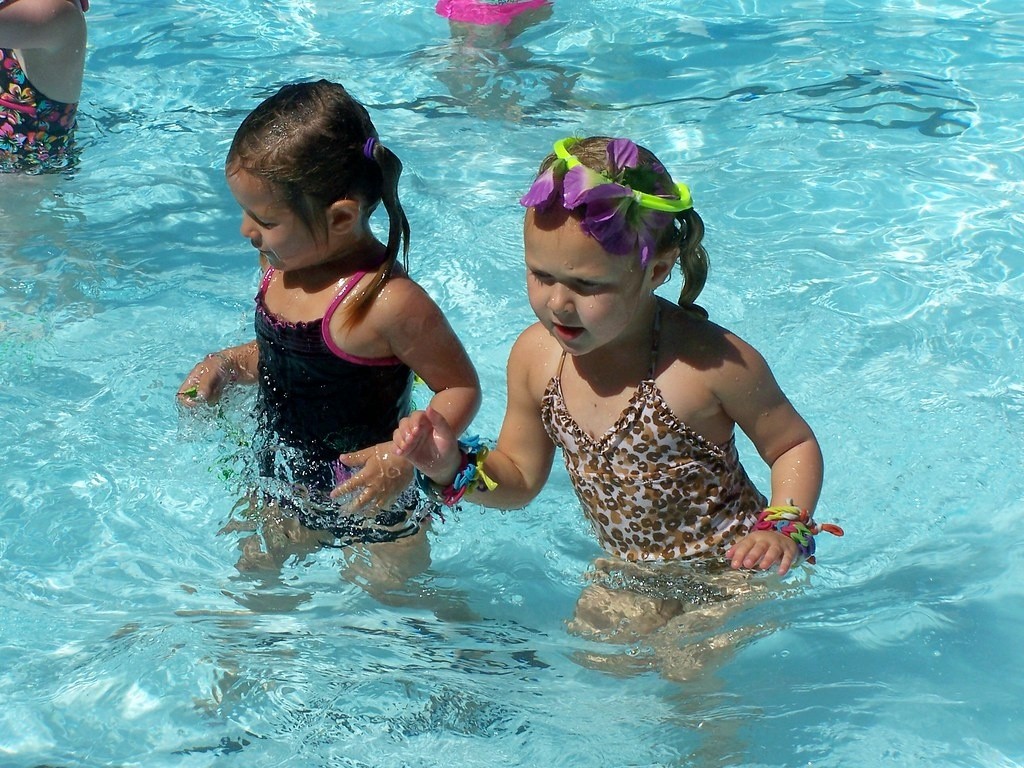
[414,434,496,506]
[746,497,843,566]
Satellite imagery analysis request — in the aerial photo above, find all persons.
[0,0,90,173]
[175,77,480,604]
[394,137,823,668]
[435,0,554,26]
